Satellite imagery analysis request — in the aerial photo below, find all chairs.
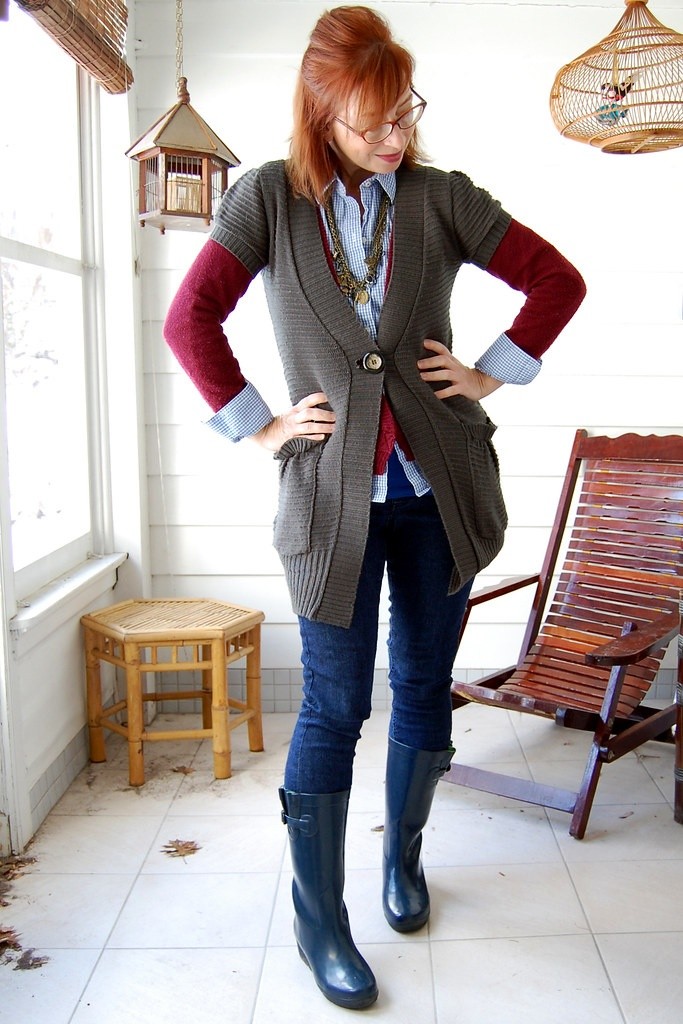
[442,430,682,840]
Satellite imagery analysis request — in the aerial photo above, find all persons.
[163,8,587,1010]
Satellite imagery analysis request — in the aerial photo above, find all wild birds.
[601,70,644,101]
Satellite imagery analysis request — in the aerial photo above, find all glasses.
[330,84,427,146]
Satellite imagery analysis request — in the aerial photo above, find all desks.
[81,599,266,790]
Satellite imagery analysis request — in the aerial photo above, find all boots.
[381,735,457,935]
[277,785,379,1010]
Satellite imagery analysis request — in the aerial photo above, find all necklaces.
[326,195,390,304]
